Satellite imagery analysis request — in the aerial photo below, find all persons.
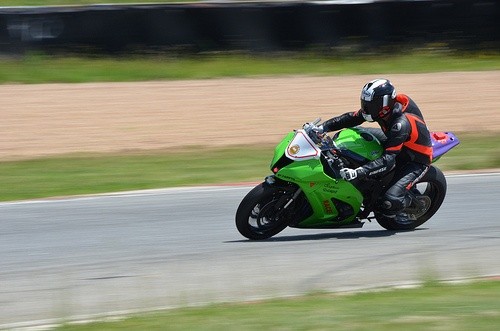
[302,78,433,218]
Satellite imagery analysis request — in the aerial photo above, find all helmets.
[360,76,397,123]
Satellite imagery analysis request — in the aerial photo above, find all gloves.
[340,167,357,182]
[302,121,325,134]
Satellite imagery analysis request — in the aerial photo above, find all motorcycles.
[235,117,461,241]
